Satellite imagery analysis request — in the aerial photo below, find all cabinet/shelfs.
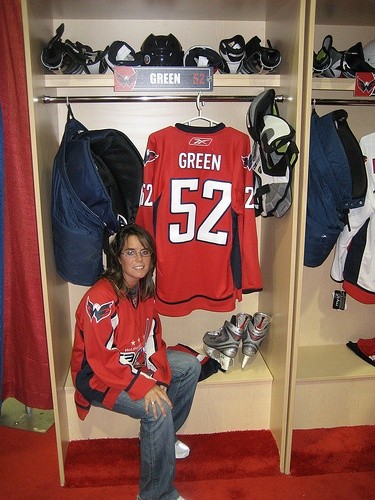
[21,0,375,486]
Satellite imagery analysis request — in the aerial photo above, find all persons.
[71,225,202,500]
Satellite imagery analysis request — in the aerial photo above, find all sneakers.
[199,312,252,372]
[240,311,272,369]
[137,430,190,459]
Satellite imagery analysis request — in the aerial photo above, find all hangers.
[182,91,218,127]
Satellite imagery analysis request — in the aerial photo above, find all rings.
[151,402,155,405]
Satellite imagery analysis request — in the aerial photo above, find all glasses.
[119,248,152,257]
